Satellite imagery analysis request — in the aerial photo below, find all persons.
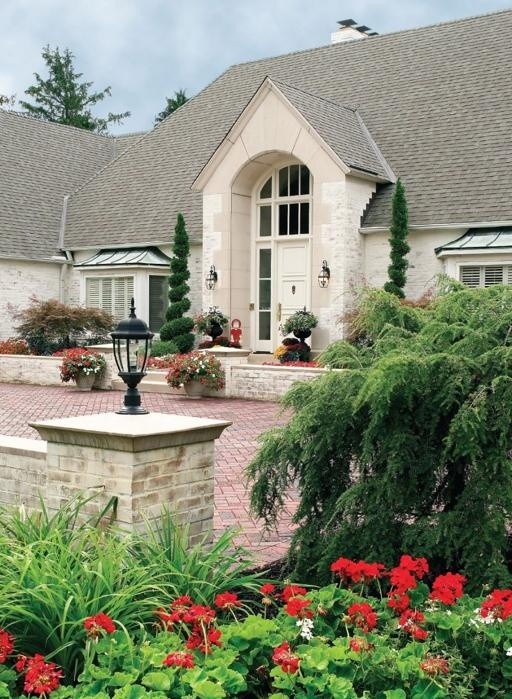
[230,318,243,344]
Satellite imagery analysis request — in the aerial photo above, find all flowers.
[277,309,320,338]
[54,345,111,384]
[194,301,231,337]
[163,348,227,392]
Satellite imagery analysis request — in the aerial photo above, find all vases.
[74,372,96,392]
[206,323,224,341]
[183,381,207,400]
[293,328,312,351]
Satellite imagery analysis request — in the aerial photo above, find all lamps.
[107,293,158,416]
[316,259,332,290]
[203,263,219,293]
